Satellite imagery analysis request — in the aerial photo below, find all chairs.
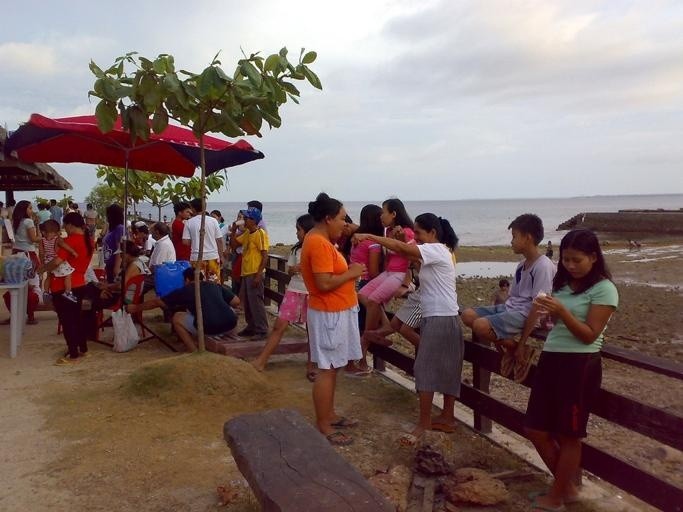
[98,274,146,341]
[56,268,106,334]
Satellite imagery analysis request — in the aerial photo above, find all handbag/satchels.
[112,310,138,352]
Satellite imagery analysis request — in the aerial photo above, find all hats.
[240,207,262,224]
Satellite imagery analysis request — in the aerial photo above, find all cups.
[236,219,245,226]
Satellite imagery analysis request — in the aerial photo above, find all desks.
[0,279,29,359]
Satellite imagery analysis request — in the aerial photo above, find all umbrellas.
[5,109,265,308]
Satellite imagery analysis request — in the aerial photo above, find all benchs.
[223,406,399,512]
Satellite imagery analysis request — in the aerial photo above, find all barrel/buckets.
[154,260,192,298]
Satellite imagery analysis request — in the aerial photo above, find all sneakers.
[62,291,78,304]
[238,329,269,340]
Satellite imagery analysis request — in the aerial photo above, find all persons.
[247,195,465,444]
[628,239,641,252]
[3,198,268,363]
[523,228,618,512]
[461,214,555,383]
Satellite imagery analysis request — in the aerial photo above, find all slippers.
[360,332,393,355]
[306,366,317,381]
[56,351,91,366]
[500,345,536,382]
[327,416,358,445]
[344,365,373,380]
[526,489,582,512]
[401,416,457,446]
